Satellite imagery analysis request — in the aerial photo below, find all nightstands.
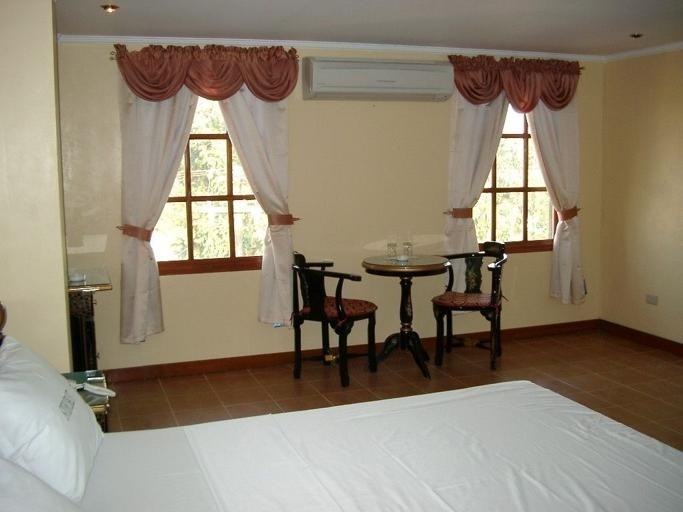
[58,370,109,433]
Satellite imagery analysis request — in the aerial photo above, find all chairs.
[430,240,508,371]
[290,249,378,389]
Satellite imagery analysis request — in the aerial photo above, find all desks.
[69,265,115,371]
[361,253,452,380]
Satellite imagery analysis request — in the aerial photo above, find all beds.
[0,302,681,509]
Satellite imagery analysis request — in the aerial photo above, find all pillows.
[0,334,105,504]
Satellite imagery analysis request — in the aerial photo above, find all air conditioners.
[299,53,458,105]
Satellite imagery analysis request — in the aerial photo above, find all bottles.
[386,240,412,262]
